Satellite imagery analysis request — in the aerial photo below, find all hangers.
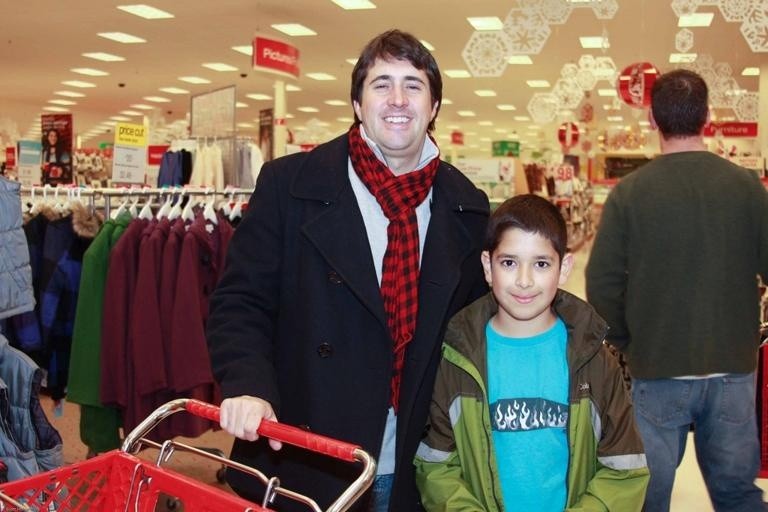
[22,184,245,225]
[166,135,217,154]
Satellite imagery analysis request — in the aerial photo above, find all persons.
[415,191,653,511]
[42,128,71,180]
[205,28,490,511]
[585,66,767,512]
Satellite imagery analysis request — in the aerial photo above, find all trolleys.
[0,398,377,512]
